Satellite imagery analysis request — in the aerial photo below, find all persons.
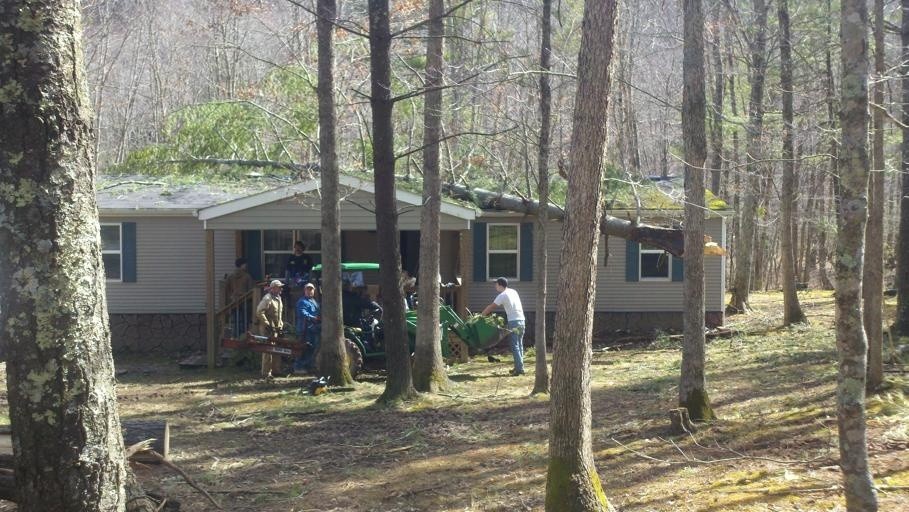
[481,277,527,376]
[293,282,322,375]
[255,280,288,379]
[224,256,256,337]
[285,240,315,278]
[341,277,379,327]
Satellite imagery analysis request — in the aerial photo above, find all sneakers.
[509,368,524,376]
[290,367,309,375]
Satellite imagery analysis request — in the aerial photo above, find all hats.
[353,281,363,289]
[305,283,314,289]
[270,280,286,288]
[492,277,508,286]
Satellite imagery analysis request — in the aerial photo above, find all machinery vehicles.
[220,262,516,381]
